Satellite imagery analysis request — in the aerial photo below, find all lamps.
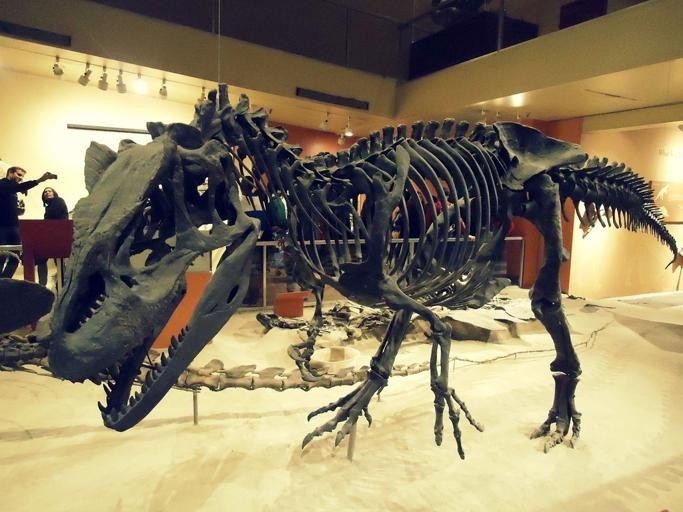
[158,78,167,95]
[114,70,126,93]
[97,66,108,90]
[197,86,206,102]
[52,55,63,75]
[480,109,520,125]
[319,111,353,146]
[77,63,92,86]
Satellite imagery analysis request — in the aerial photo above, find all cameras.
[49,174,58,180]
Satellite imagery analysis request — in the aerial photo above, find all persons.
[267,187,477,241]
[38,187,68,290]
[0,165,58,278]
